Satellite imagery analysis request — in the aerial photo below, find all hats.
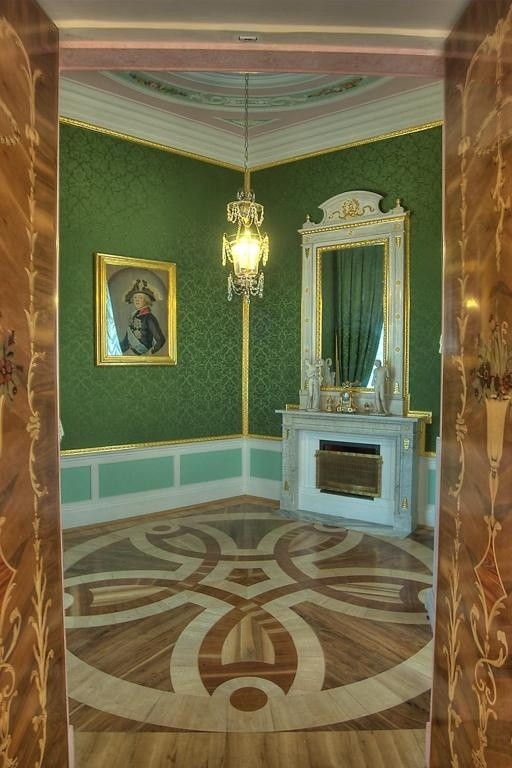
[122,278,162,304]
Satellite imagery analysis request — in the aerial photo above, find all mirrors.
[297,190,410,416]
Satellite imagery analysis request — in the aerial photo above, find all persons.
[371,359,390,413]
[305,357,325,409]
[120,278,166,356]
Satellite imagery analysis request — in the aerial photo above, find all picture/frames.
[92,251,178,367]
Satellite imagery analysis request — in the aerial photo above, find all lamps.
[221,72,270,304]
